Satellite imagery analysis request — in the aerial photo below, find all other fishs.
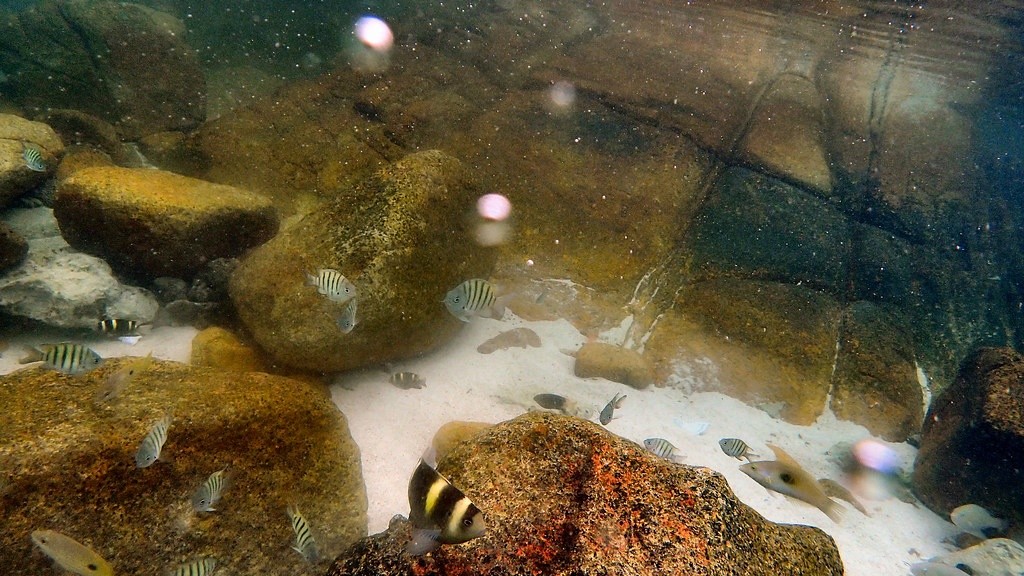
[2,136,1006,575]
